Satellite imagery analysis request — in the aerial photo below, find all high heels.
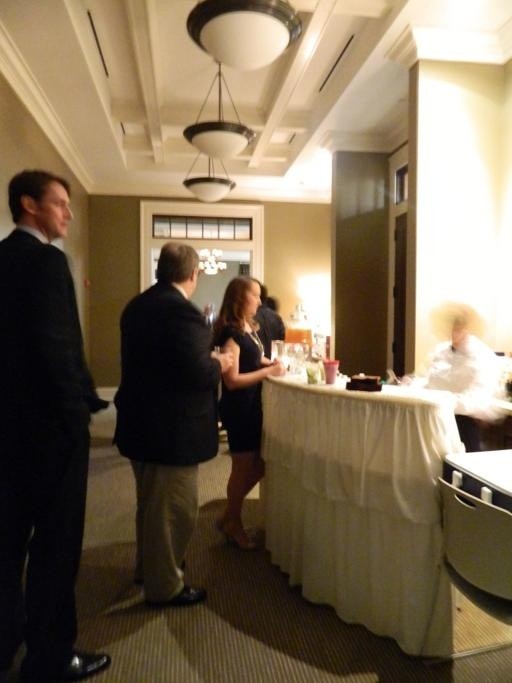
[218,517,254,549]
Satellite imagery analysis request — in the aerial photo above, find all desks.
[442,447,512,510]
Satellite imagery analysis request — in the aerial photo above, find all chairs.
[410,476,512,682]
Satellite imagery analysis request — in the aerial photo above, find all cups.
[213,345,234,356]
[269,337,340,387]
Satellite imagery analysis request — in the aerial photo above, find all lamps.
[182,150,238,203]
[182,56,254,162]
[187,1,303,72]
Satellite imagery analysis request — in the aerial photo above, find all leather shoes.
[23,649,110,677]
[147,584,207,607]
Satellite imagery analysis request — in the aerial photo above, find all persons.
[0,169,112,682]
[391,304,506,490]
[199,273,287,551]
[119,243,232,610]
